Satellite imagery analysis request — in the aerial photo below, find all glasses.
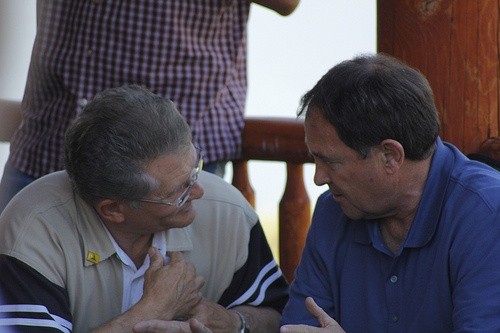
[134,145,205,208]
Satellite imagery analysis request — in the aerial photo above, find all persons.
[1,0,301,214]
[278,52,500,333]
[0,83,290,333]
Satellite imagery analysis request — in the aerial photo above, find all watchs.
[227,308,251,333]
